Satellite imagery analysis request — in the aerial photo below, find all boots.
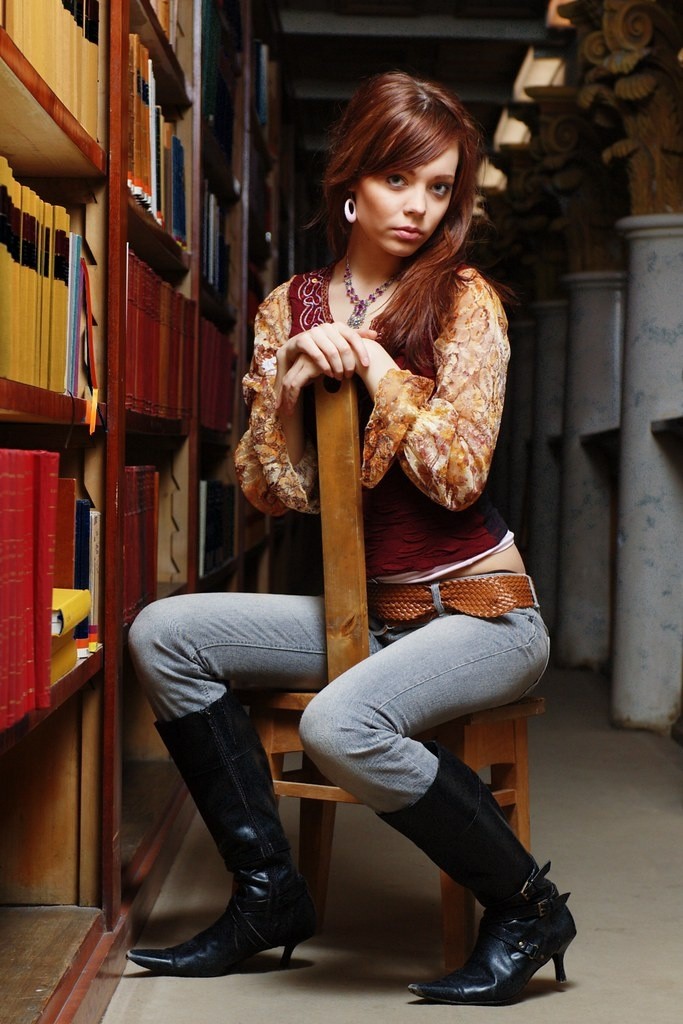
[124,688,318,975]
[374,740,576,1005]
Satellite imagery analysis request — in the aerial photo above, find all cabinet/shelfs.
[0,0,308,1024]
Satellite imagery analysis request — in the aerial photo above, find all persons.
[123,73,578,1006]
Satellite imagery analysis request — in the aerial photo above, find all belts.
[366,574,535,629]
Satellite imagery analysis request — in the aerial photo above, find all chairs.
[228,370,549,967]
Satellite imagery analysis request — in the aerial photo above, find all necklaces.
[344,251,400,330]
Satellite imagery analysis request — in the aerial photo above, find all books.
[0,1,243,731]
